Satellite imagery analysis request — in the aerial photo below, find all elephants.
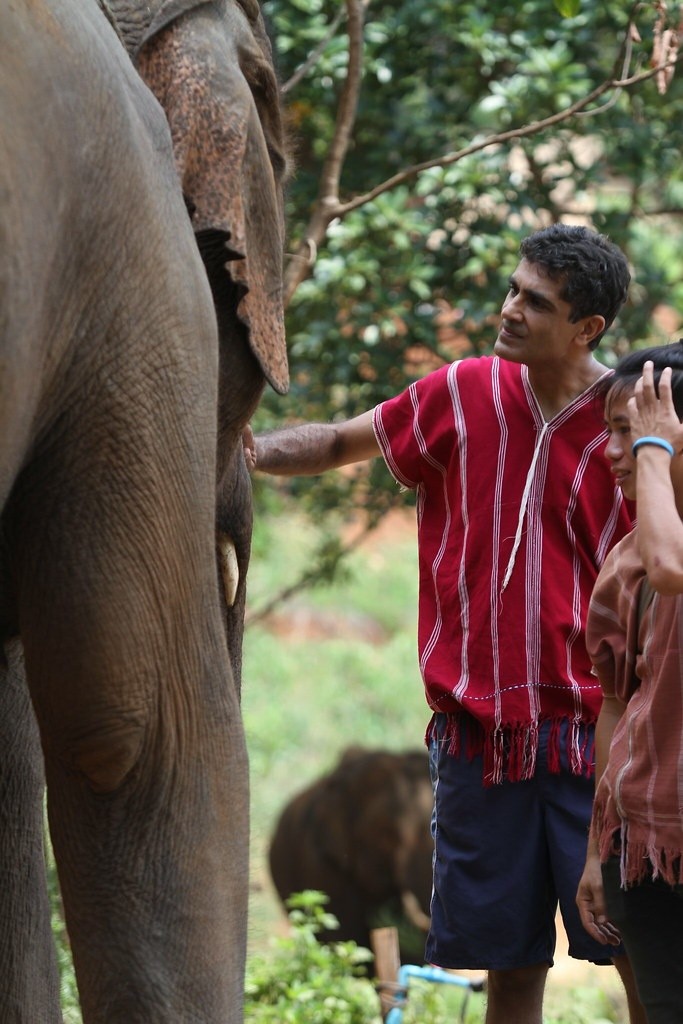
[0,0,289,1024]
[269,749,439,967]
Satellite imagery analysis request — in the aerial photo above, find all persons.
[244,223,632,1023]
[573,341,683,1024]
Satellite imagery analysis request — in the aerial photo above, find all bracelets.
[633,435,675,456]
